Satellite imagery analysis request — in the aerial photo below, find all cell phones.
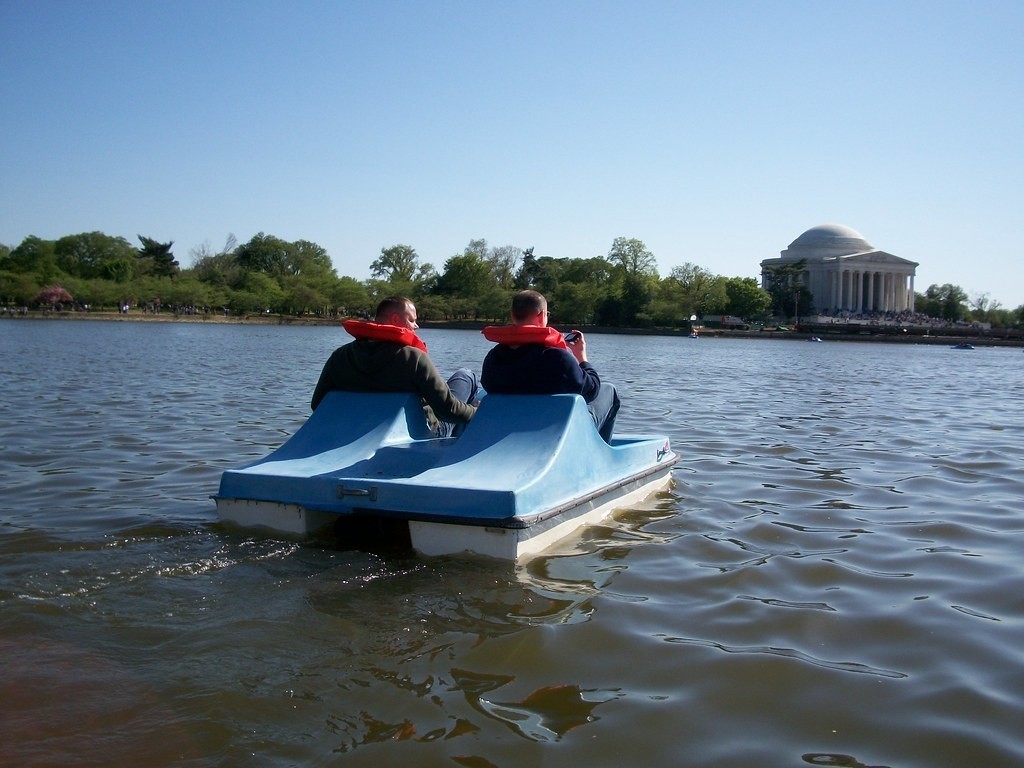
[564,333,580,342]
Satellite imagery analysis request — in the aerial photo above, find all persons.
[311,297,481,437]
[812,309,978,346]
[480,290,621,446]
[3,303,229,319]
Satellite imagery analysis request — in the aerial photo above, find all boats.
[209,387,682,578]
[950,343,975,350]
[688,329,699,338]
[805,337,824,343]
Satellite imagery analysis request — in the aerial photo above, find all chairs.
[210,391,672,519]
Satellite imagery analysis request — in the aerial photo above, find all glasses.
[537,311,550,317]
